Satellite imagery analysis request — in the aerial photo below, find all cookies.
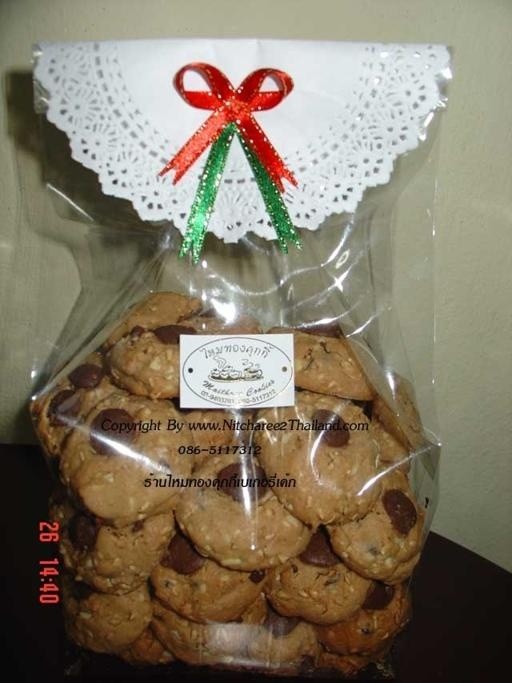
[32,289,426,675]
